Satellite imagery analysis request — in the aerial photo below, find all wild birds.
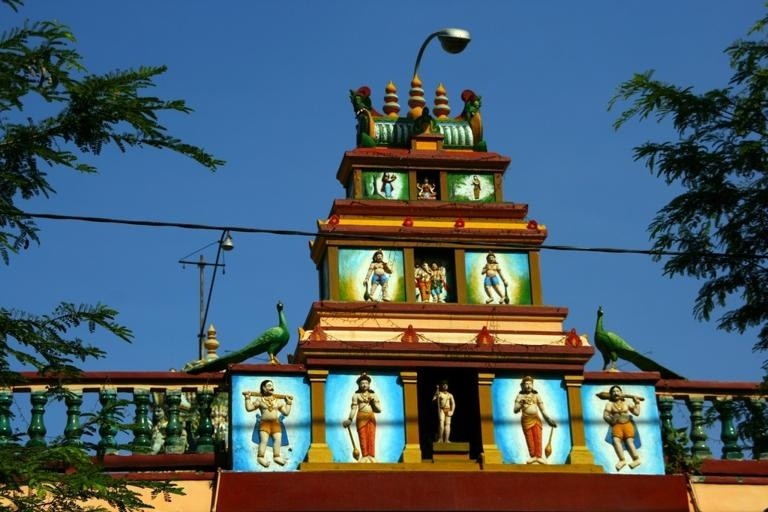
[594,305,687,382]
[185,300,292,375]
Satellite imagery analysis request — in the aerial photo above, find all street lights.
[409,28,470,79]
[178,227,234,360]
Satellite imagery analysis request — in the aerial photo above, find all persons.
[432,380,456,444]
[595,385,645,470]
[242,377,294,468]
[163,391,197,453]
[513,376,557,465]
[469,175,484,200]
[379,171,397,198]
[478,252,512,306]
[360,249,395,304]
[342,371,381,463]
[414,258,451,307]
[416,178,438,202]
[151,403,169,456]
